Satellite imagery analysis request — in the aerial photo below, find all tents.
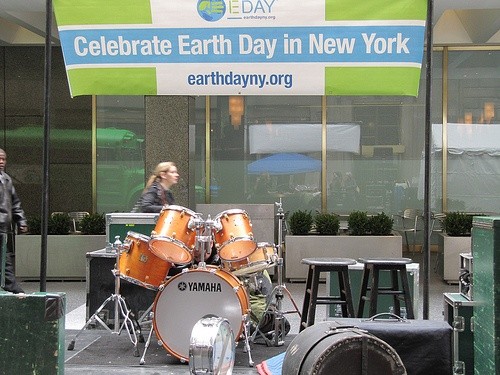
[418,122,500,217]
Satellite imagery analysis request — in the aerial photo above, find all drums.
[149,205,206,265]
[111,230,172,291]
[212,208,258,262]
[221,242,279,276]
[149,265,248,363]
[189,317,235,375]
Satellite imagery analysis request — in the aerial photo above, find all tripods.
[249,198,307,350]
[67,236,144,357]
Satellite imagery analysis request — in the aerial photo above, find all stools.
[358,256,414,321]
[298,258,357,332]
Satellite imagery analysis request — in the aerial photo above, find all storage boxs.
[325,260,421,322]
[442,291,473,375]
[0,291,65,375]
[458,252,472,301]
[85,247,176,328]
[470,215,500,375]
[104,212,160,248]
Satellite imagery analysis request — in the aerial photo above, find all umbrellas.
[246,153,322,175]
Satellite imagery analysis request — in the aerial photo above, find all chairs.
[51,212,90,234]
[390,208,444,271]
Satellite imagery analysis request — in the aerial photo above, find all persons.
[0,149,28,294]
[328,170,361,207]
[136,161,180,213]
[255,173,272,204]
